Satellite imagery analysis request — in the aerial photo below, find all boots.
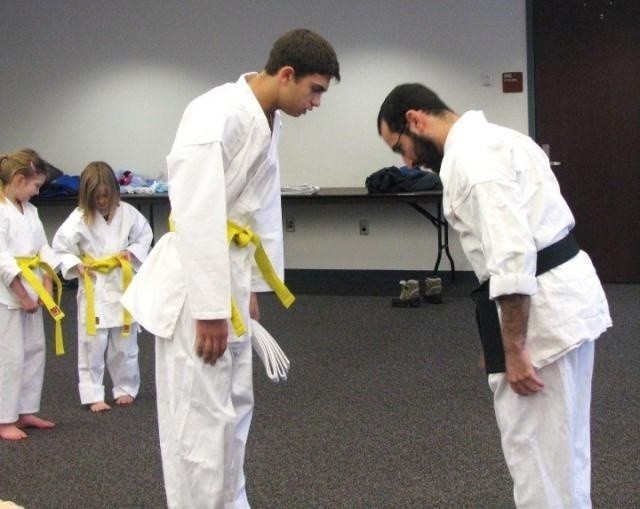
[423,277,443,304]
[391,279,421,308]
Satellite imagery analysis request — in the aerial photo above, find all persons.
[0,149,65,440]
[378,83,613,509]
[51,162,153,412]
[116,30,342,509]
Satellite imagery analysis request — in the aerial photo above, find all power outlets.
[286,215,295,233]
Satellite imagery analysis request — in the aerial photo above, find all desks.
[29,186,456,286]
